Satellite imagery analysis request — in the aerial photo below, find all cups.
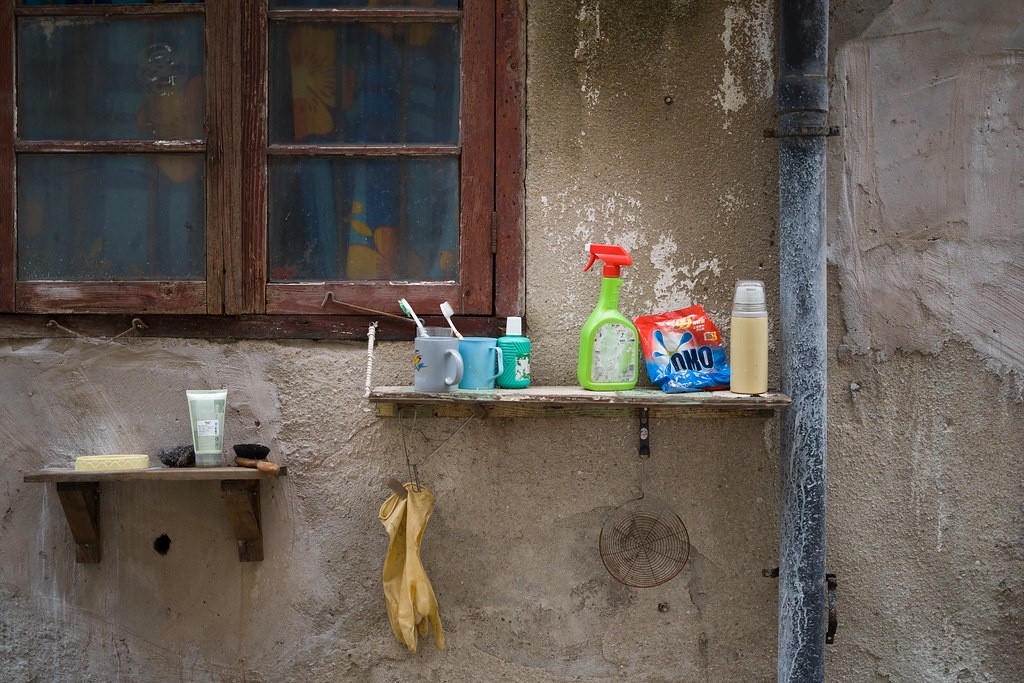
[414,336,464,393]
[458,337,504,390]
[417,327,453,338]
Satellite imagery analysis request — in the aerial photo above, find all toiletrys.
[183,387,229,468]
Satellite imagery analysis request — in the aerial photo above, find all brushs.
[233,444,279,474]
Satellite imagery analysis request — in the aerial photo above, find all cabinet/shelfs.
[22,384,792,564]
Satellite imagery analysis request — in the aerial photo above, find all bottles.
[730,279,770,393]
[499,317,531,388]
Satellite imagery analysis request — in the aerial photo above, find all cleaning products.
[632,303,731,394]
[576,241,641,390]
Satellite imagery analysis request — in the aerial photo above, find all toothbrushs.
[440,300,464,340]
[397,297,430,338]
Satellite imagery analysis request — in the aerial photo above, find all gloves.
[379,482,445,654]
